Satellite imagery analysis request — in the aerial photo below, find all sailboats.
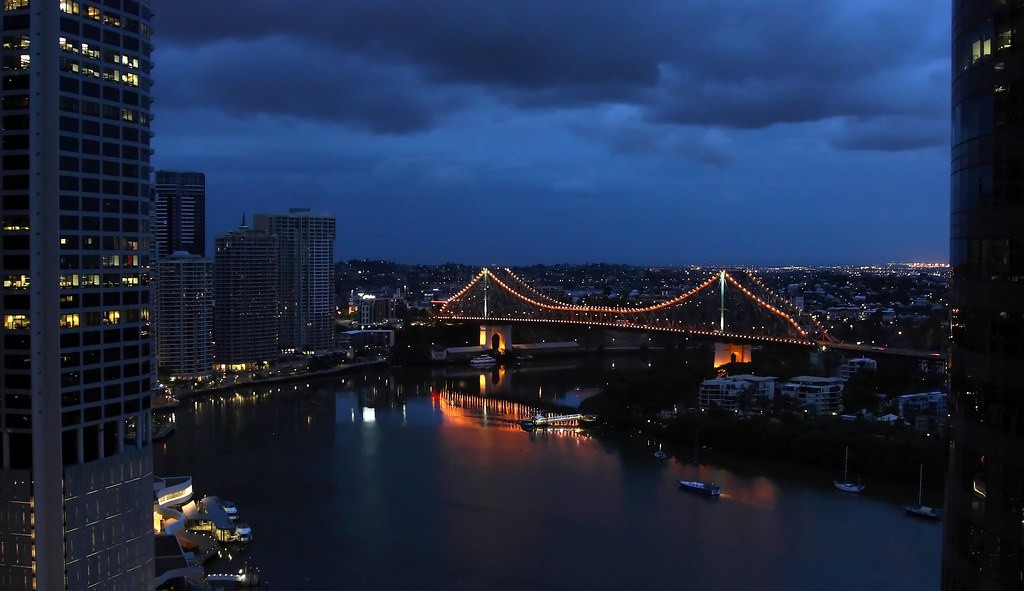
[833,446,866,492]
[903,463,937,518]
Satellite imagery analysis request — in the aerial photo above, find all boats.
[234,523,253,543]
[655,451,667,459]
[517,354,533,360]
[222,505,240,520]
[679,480,720,496]
[470,354,497,363]
[520,418,534,428]
